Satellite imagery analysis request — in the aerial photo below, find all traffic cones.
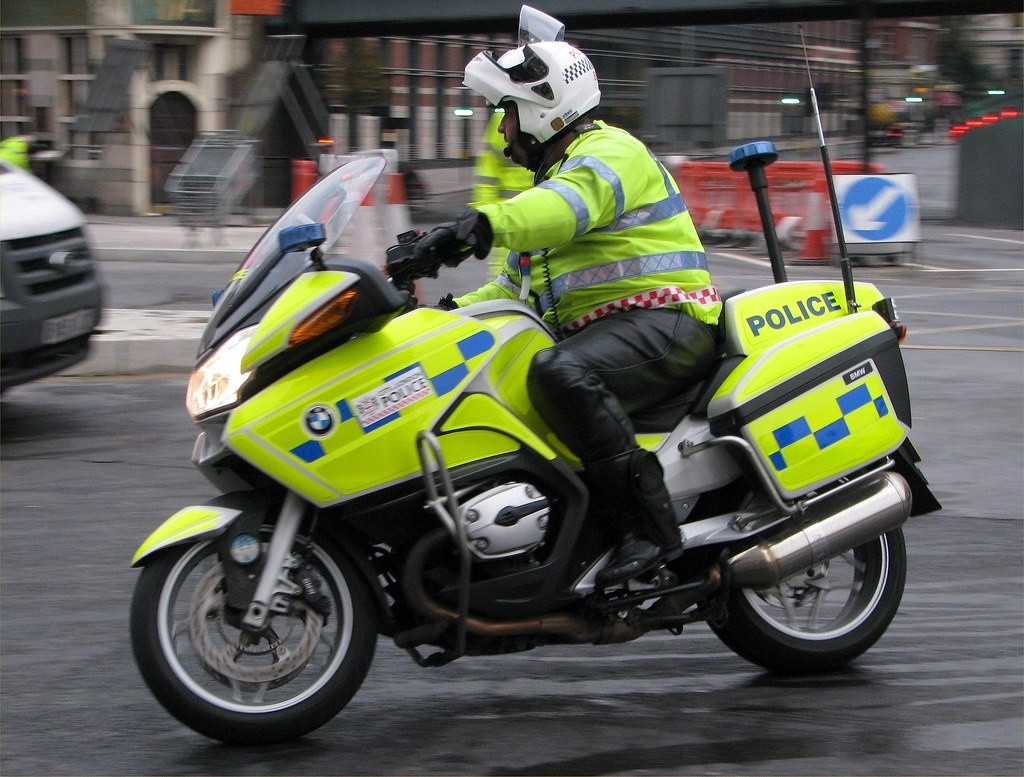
[385,172,420,304]
[357,179,385,272]
[797,179,831,260]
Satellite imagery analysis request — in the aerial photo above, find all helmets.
[459,6,600,147]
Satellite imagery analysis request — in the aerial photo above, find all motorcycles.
[129,87,944,746]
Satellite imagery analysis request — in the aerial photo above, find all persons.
[0,134,35,174]
[414,41,723,589]
[468,109,536,283]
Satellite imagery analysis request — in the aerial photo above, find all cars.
[863,124,903,144]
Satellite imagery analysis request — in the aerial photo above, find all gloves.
[391,212,490,274]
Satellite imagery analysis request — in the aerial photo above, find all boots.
[588,448,682,589]
